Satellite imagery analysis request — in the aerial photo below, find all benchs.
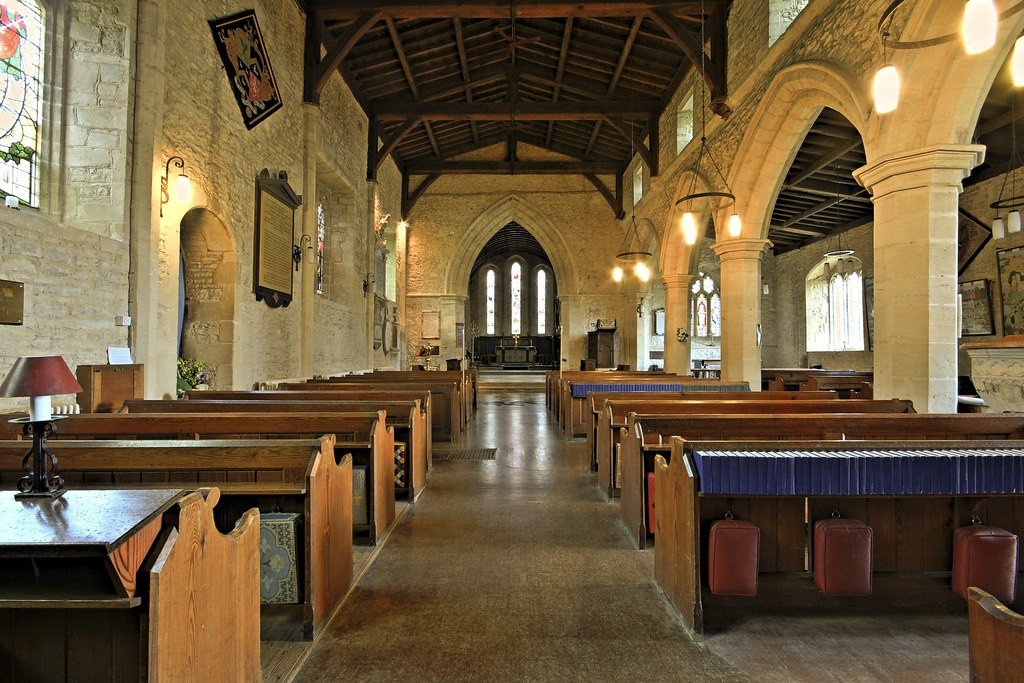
[0,365,1024,683]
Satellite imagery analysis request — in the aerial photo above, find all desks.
[495,345,536,362]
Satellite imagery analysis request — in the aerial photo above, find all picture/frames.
[958,277,995,337]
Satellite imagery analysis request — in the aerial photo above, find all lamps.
[159,156,189,218]
[293,234,314,271]
[989,91,1024,240]
[362,272,377,294]
[612,68,654,281]
[0,355,83,499]
[674,1,740,243]
[760,277,769,295]
[637,297,645,318]
[823,162,855,276]
[871,0,1024,115]
